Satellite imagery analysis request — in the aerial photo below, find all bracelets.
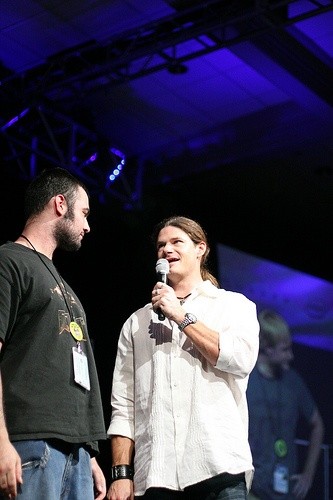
[110,464,134,482]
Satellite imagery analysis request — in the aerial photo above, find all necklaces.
[18,234,83,343]
[254,363,290,457]
[176,293,192,304]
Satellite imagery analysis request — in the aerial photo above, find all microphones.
[156,259,170,321]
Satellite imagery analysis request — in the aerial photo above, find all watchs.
[179,313,197,333]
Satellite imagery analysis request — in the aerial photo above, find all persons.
[0,165,108,500]
[246,306,324,500]
[105,216,261,500]
